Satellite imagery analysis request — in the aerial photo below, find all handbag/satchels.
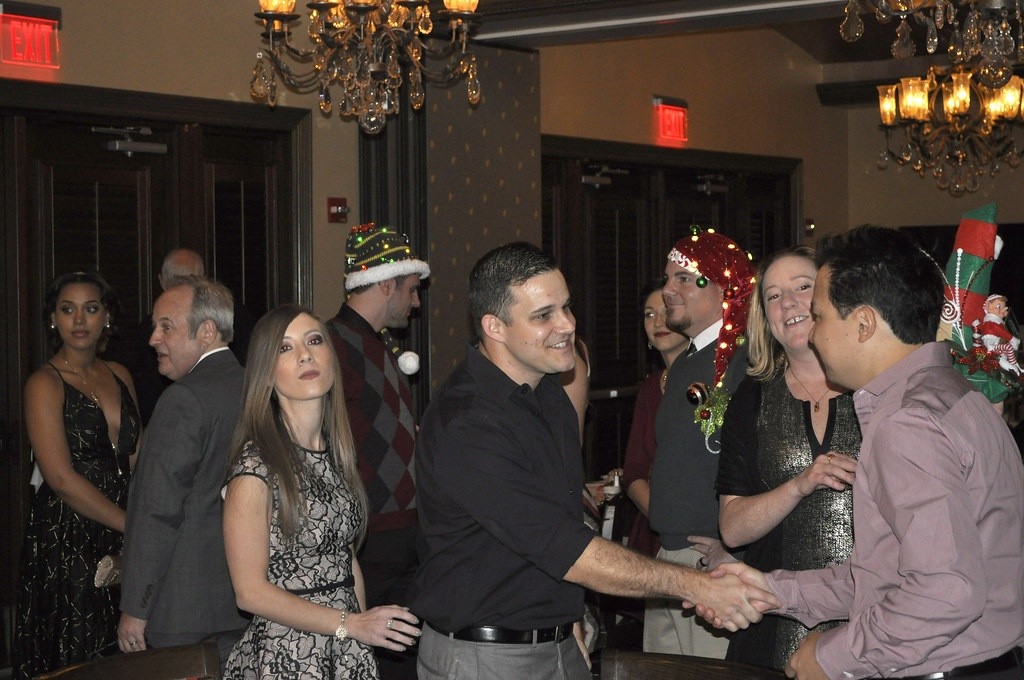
[94,554,124,587]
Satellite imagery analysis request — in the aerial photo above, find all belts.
[289,574,356,596]
[425,618,574,644]
[857,644,1024,680]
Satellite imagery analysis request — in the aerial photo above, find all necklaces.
[64,359,100,409]
[663,375,667,382]
[790,367,832,412]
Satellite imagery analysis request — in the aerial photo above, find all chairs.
[30,641,222,680]
[599,647,794,680]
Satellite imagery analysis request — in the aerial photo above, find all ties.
[686,342,696,358]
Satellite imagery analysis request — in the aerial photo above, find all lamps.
[837,0,1024,202]
[249,0,484,135]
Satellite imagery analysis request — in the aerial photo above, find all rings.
[828,454,836,463]
[700,556,707,567]
[130,641,137,645]
[387,619,394,630]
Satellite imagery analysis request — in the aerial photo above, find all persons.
[545,334,599,654]
[117,278,254,654]
[325,224,430,680]
[981,294,1024,377]
[221,304,423,680]
[712,248,862,672]
[409,242,781,680]
[140,248,206,391]
[13,272,143,680]
[682,225,1024,680]
[613,278,690,640]
[648,231,756,661]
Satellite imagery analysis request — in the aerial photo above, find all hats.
[343,224,431,374]
[667,229,758,439]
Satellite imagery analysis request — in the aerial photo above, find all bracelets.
[336,610,349,642]
